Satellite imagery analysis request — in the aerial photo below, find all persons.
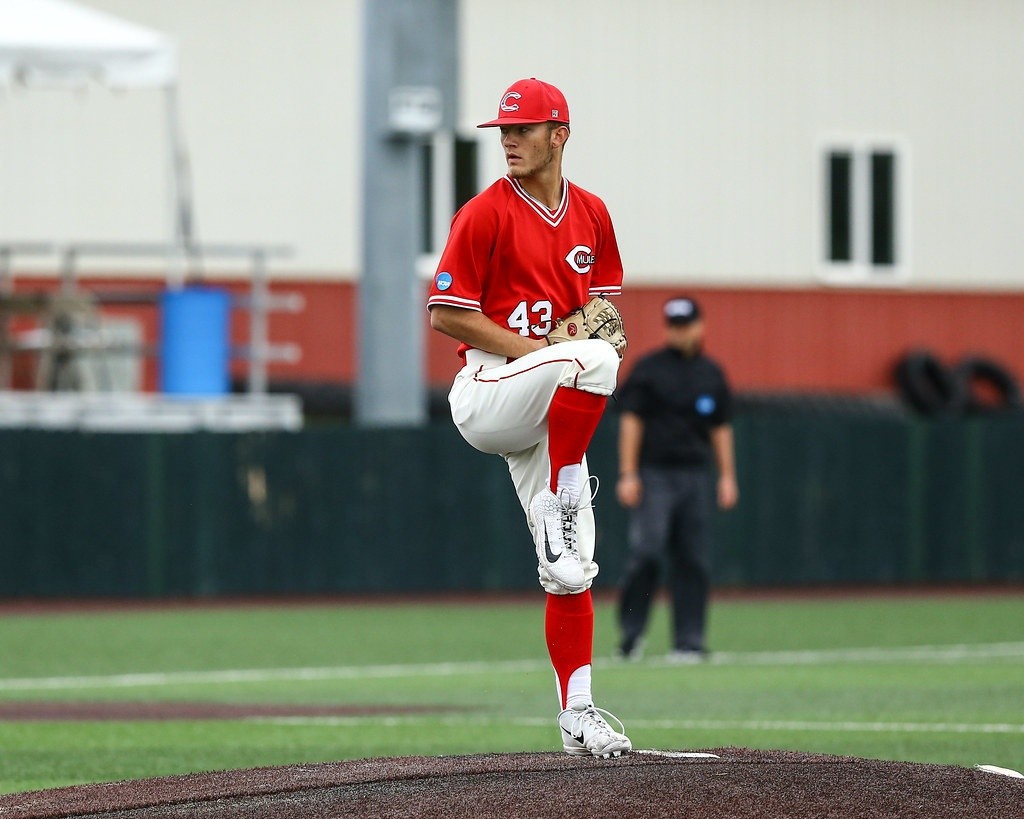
[619,297,737,666]
[428,79,631,761]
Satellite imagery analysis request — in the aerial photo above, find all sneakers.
[529,475,600,589]
[557,702,631,758]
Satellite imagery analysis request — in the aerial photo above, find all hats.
[663,297,698,324]
[477,78,569,128]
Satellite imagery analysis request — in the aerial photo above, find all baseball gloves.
[533,289,629,365]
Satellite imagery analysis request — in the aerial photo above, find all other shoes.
[671,642,711,660]
[617,632,643,660]
